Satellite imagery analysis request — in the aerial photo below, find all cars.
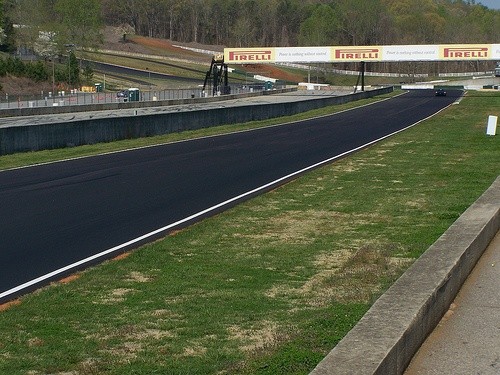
[435,89,446,96]
[116,89,128,97]
